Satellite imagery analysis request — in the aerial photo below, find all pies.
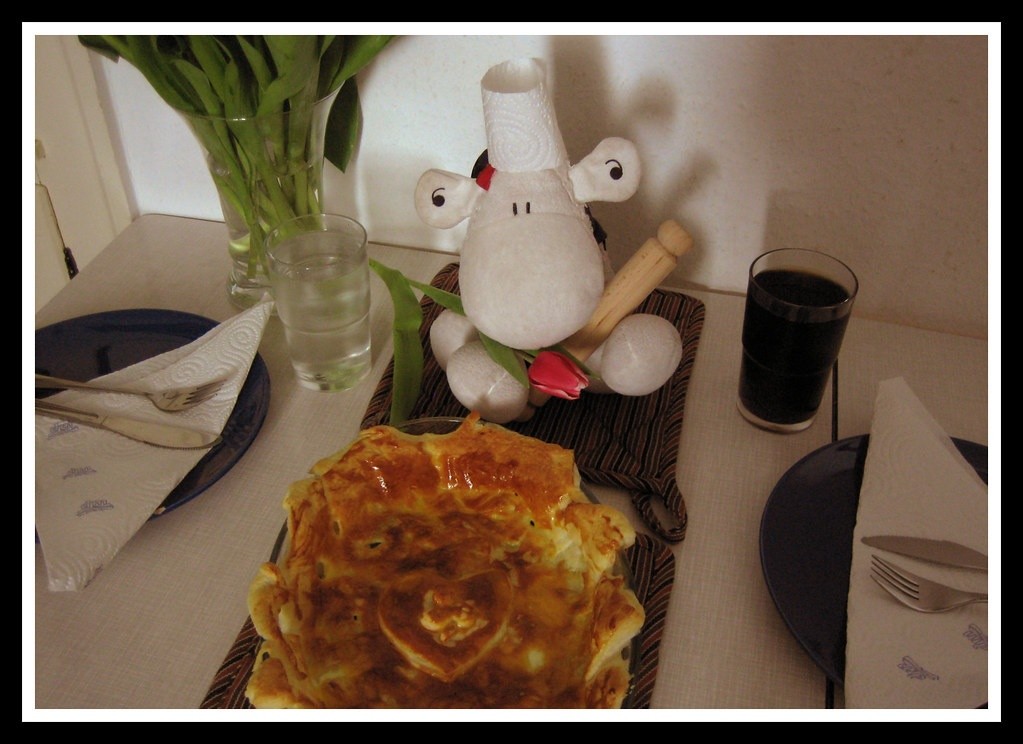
[244,414,646,709]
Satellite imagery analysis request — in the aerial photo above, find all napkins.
[843,378,989,710]
[35,290,276,593]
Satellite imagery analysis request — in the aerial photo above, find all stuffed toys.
[413,57,682,424]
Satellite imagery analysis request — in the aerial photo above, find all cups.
[266,216,371,395]
[737,248,858,434]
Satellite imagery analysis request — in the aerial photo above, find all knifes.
[863,536,987,571]
[35,400,225,449]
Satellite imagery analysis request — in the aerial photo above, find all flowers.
[80,35,595,433]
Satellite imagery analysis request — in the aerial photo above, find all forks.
[870,554,987,614]
[36,373,228,411]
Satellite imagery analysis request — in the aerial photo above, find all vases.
[178,76,346,317]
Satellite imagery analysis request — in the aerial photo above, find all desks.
[34,214,988,709]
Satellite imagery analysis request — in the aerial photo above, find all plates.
[267,414,632,706]
[37,308,271,521]
[761,435,988,709]
